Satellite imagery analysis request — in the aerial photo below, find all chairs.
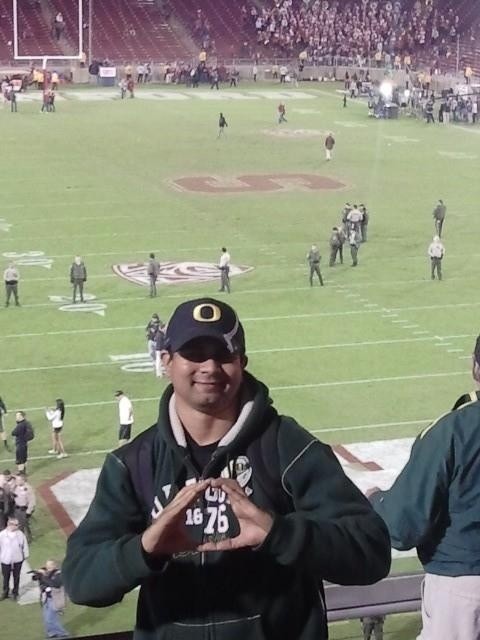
[1,0,480,81]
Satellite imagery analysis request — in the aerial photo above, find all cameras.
[32,567,47,581]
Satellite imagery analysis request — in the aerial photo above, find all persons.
[215,246,232,294]
[1,0,479,141]
[1,397,70,639]
[328,202,369,268]
[146,312,170,380]
[307,244,324,287]
[432,199,446,240]
[324,132,336,161]
[147,252,161,298]
[61,298,392,640]
[3,261,22,309]
[360,615,386,640]
[364,335,480,639]
[428,236,445,280]
[113,389,135,449]
[70,255,88,305]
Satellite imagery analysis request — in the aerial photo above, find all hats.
[167,298,245,354]
